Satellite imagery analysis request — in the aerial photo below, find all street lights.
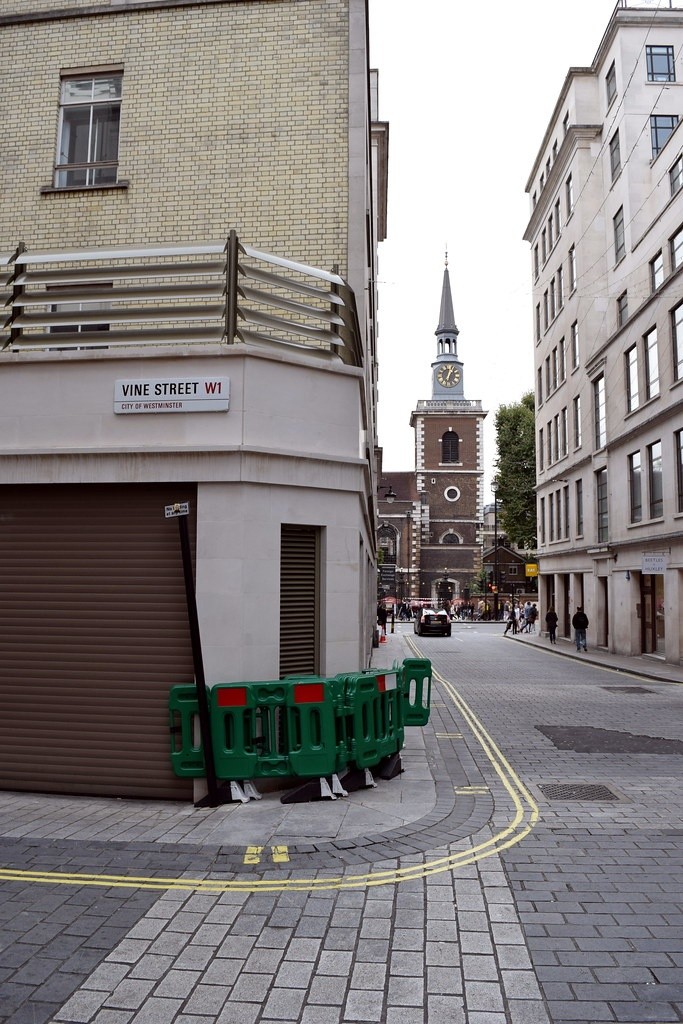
[489,475,504,620]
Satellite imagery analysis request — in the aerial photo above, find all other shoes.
[583,646,587,651]
[575,649,581,653]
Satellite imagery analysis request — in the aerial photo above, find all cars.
[411,607,454,637]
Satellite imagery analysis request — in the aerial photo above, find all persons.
[376,597,539,637]
[572,606,589,652]
[545,606,558,645]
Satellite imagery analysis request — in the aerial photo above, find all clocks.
[437,364,461,388]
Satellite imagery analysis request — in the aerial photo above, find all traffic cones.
[378,623,388,643]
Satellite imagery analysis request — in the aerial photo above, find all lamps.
[377,484,395,504]
